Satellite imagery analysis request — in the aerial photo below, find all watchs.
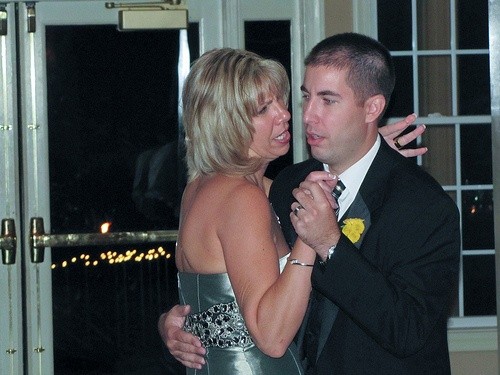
[326,245,337,262]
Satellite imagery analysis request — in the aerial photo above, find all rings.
[293,206,304,216]
[394,138,402,149]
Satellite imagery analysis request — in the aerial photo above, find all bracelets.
[287,257,314,267]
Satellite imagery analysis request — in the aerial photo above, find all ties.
[329,180,348,226]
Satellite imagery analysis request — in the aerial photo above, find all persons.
[157,32,461,375]
[176,49,428,374]
